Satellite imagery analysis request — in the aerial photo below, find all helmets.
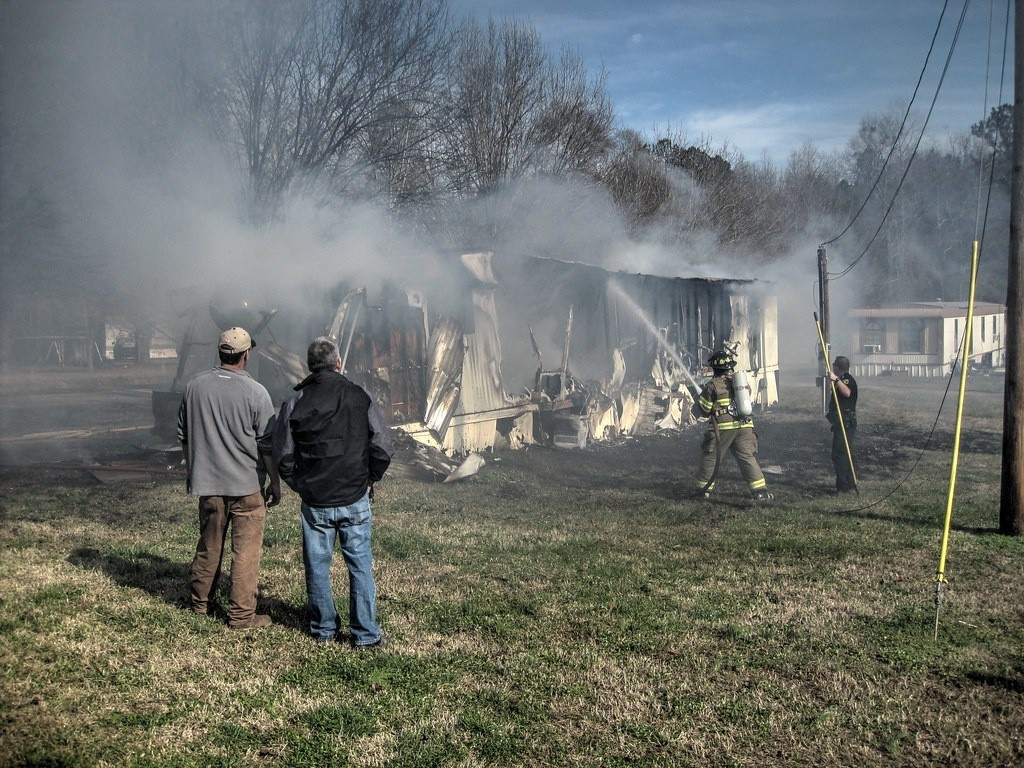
[708,350,737,370]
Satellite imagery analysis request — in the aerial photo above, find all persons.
[826,356,858,492]
[176,326,281,629]
[272,336,392,647]
[696,350,774,501]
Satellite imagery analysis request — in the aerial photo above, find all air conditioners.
[862,345,876,353]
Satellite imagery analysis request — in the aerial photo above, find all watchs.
[836,378,839,382]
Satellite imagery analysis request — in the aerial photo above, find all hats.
[218,327,256,354]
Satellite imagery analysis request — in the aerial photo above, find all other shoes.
[318,636,331,642]
[693,487,709,498]
[358,638,382,648]
[232,614,272,629]
[755,492,774,500]
